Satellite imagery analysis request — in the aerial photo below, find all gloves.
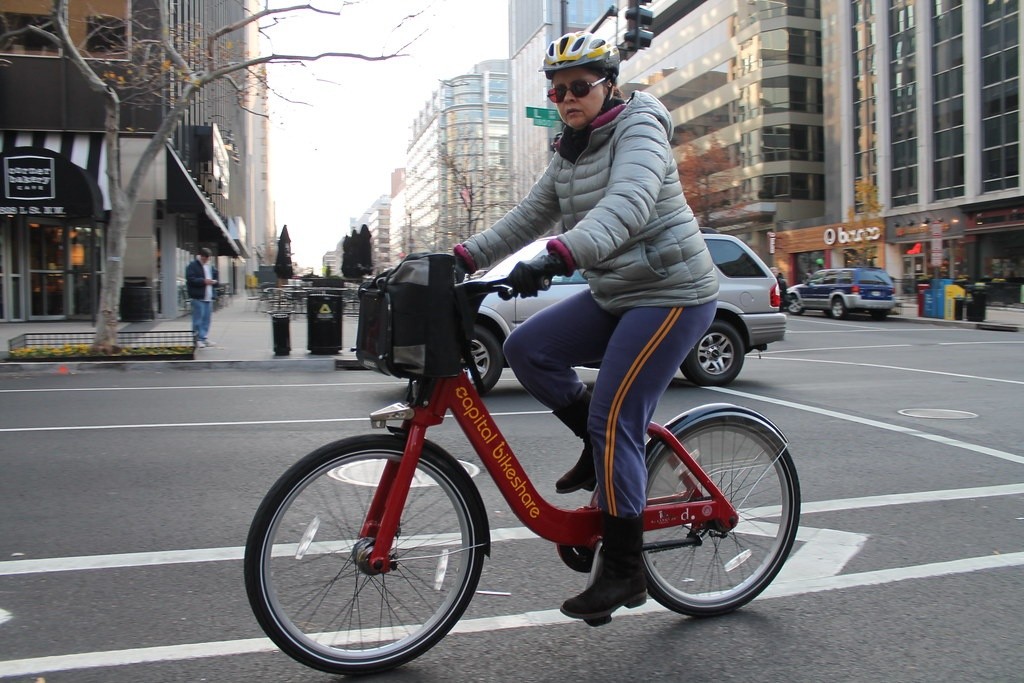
[447,245,477,283]
[508,240,574,299]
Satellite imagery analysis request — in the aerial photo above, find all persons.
[449,31,719,620]
[817,264,823,270]
[186,248,218,348]
[769,267,787,313]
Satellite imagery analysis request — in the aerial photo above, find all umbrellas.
[340,224,373,278]
[274,225,293,279]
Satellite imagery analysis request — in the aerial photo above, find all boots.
[561,515,648,619]
[555,389,600,494]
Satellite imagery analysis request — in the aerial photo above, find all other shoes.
[204,339,216,347]
[196,340,206,347]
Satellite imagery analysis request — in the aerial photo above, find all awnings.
[167,142,239,258]
[0,145,103,220]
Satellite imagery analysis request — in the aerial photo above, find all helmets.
[538,31,620,81]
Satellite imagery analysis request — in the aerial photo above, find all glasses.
[548,75,608,103]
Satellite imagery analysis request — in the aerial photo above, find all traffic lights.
[624,0,655,49]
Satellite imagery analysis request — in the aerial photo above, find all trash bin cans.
[964,285,989,322]
[307,294,343,355]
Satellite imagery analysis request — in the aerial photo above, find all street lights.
[405,209,413,254]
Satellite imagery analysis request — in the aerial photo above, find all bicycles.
[242,276,800,683]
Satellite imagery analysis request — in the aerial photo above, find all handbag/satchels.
[355,252,474,378]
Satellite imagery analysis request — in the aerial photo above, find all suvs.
[463,227,788,386]
[784,267,897,319]
